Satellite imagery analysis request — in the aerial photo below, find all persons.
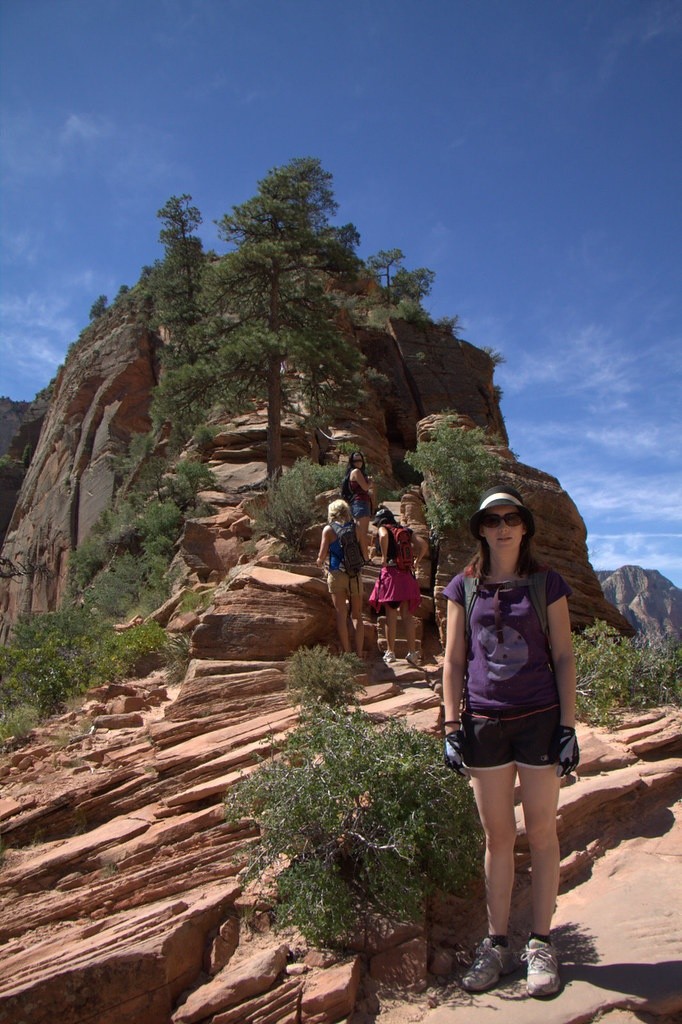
[438,483,580,997]
[316,498,375,661]
[343,450,379,569]
[368,505,430,667]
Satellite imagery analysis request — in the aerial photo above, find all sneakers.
[525,939,560,996]
[405,653,418,663]
[462,938,520,991]
[382,651,395,662]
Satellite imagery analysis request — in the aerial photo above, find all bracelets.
[364,559,372,563]
[443,720,461,726]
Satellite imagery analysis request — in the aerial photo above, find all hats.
[470,485,534,540]
[376,509,394,518]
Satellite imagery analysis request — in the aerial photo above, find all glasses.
[353,459,362,463]
[480,512,522,528]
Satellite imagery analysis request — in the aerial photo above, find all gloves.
[557,726,580,777]
[445,731,469,776]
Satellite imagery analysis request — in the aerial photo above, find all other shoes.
[365,559,374,565]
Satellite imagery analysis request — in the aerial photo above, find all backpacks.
[341,477,360,504]
[383,524,414,567]
[329,521,364,572]
